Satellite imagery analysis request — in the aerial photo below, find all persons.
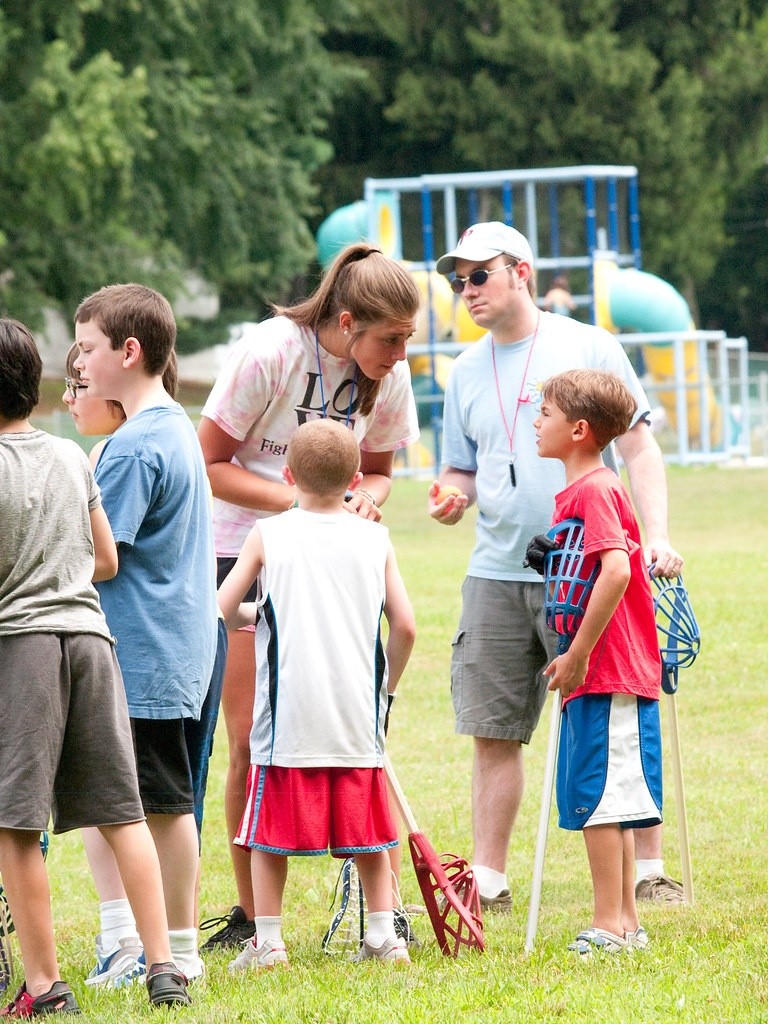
[199,245,420,957]
[62,342,228,987]
[544,274,576,318]
[73,283,217,988]
[218,419,416,969]
[430,222,691,915]
[0,317,192,1014]
[533,368,662,957]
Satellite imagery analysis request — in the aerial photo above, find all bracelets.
[354,488,375,505]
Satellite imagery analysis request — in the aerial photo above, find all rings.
[671,568,677,577]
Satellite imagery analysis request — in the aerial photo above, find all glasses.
[64,378,89,399]
[449,263,513,294]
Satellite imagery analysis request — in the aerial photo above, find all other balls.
[437,485,465,515]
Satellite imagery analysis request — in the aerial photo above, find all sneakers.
[394,910,420,946]
[227,933,290,967]
[85,935,152,988]
[407,885,512,916]
[174,954,205,982]
[5,979,79,1015]
[350,936,411,964]
[148,963,194,1007]
[566,928,651,954]
[197,907,255,953]
[636,876,685,904]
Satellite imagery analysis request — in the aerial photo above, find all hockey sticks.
[522,519,600,953]
[647,563,700,908]
[381,753,486,958]
[0,832,49,938]
[0,901,15,992]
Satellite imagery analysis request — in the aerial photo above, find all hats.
[435,220,535,265]
